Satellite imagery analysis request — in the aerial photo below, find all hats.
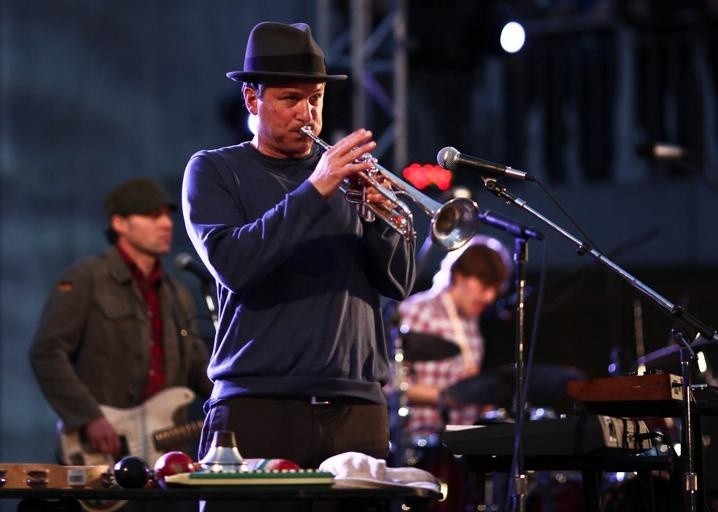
[226,21,348,82]
[107,175,181,214]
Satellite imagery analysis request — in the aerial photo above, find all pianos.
[162,469,335,483]
[443,409,665,474]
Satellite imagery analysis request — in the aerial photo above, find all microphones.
[175,252,215,290]
[675,332,696,358]
[478,208,545,240]
[436,147,534,180]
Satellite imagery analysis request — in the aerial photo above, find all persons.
[181,21,416,512]
[395,245,509,440]
[27,177,215,467]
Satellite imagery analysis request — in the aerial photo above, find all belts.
[246,389,375,408]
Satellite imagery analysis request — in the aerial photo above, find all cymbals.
[434,362,593,407]
[400,332,461,360]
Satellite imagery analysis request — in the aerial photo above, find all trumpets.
[301,126,479,250]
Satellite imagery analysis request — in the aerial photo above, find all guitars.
[58,387,206,512]
